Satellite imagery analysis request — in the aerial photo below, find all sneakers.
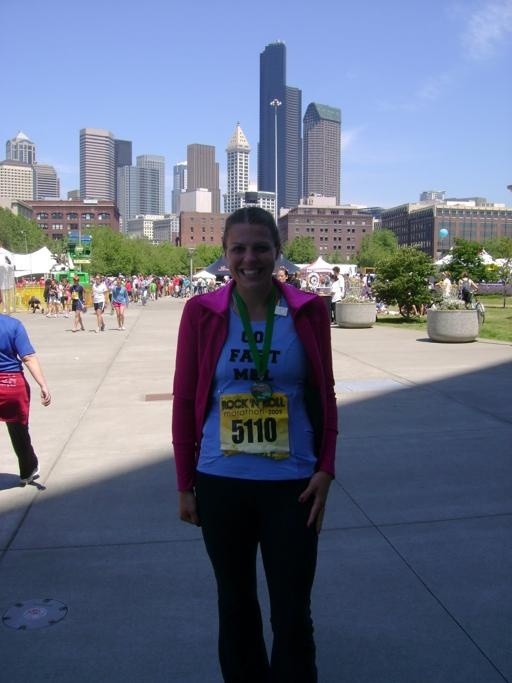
[20,462,41,485]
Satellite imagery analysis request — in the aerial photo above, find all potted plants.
[425,299,481,343]
[334,296,377,328]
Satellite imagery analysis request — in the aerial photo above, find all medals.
[251,377,273,401]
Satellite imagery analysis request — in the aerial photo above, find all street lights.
[270,98,282,227]
[21,230,28,254]
[188,248,196,297]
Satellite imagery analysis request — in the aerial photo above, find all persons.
[17,261,233,334]
[399,270,481,320]
[276,265,387,325]
[0,288,52,486]
[171,207,339,683]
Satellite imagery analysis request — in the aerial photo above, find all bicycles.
[398,301,415,318]
[465,288,485,324]
